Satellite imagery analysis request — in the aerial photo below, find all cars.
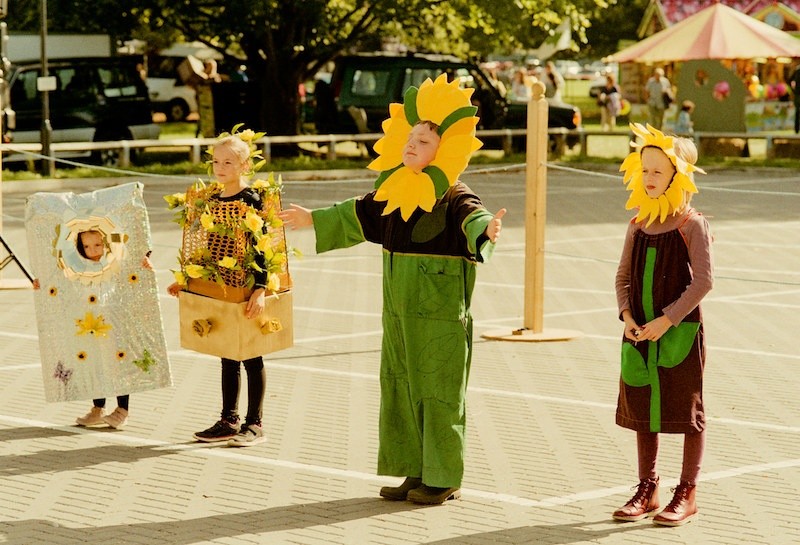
[484,54,607,81]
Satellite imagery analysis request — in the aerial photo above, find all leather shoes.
[653,482,698,526]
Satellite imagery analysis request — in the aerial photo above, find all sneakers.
[612,476,660,521]
[194,419,241,442]
[228,423,266,446]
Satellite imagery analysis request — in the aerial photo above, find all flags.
[534,16,572,61]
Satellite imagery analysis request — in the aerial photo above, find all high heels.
[381,476,422,500]
[407,483,461,504]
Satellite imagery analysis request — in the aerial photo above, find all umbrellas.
[600,2,800,61]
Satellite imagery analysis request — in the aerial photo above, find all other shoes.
[102,407,128,430]
[77,407,107,425]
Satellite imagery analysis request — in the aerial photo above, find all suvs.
[312,49,584,163]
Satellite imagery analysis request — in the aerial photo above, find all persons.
[675,100,697,134]
[642,67,673,130]
[276,71,508,508]
[612,120,714,526]
[70,231,129,427]
[784,69,800,134]
[172,58,221,140]
[488,61,622,124]
[168,122,268,448]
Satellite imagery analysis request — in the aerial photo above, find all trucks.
[0,30,200,172]
